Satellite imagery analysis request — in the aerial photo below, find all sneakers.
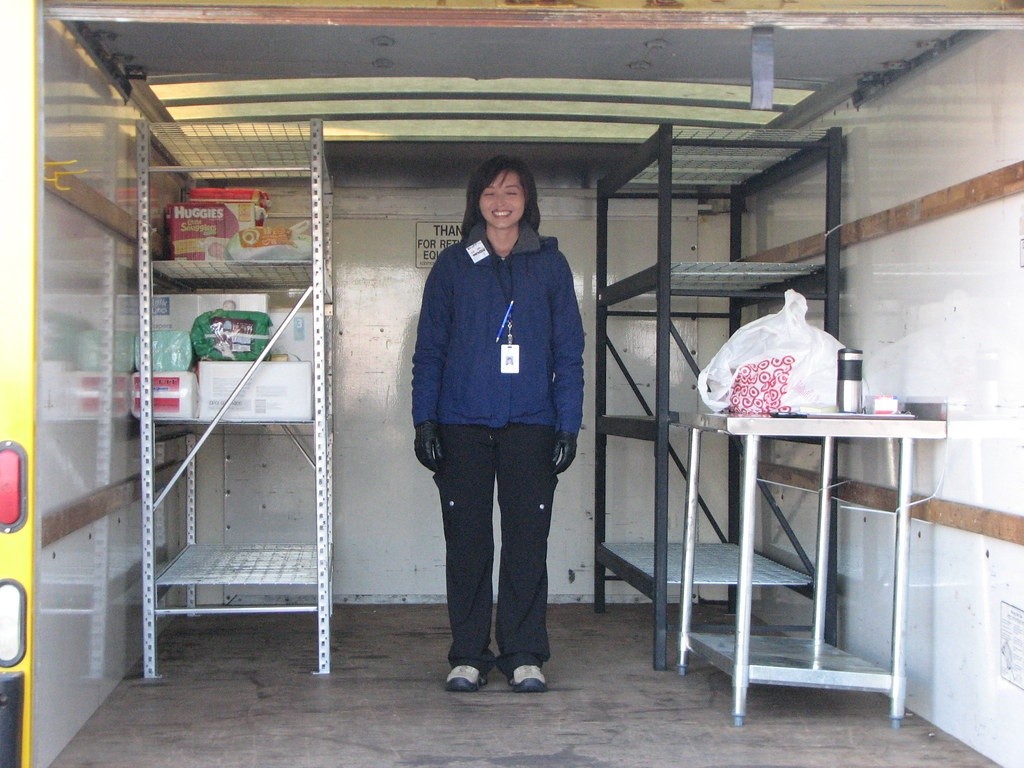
[512,664,548,693]
[446,665,488,692]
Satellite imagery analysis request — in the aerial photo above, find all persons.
[411,155,586,693]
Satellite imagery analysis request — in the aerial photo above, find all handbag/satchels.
[696,288,868,415]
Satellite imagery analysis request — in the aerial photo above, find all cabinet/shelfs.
[133,119,333,679]
[596,123,841,670]
[676,411,945,729]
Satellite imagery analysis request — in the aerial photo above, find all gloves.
[413,421,444,474]
[550,430,577,477]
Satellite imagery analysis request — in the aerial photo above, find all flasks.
[836,347,864,413]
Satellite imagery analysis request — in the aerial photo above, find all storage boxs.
[167,186,270,261]
[130,293,315,424]
